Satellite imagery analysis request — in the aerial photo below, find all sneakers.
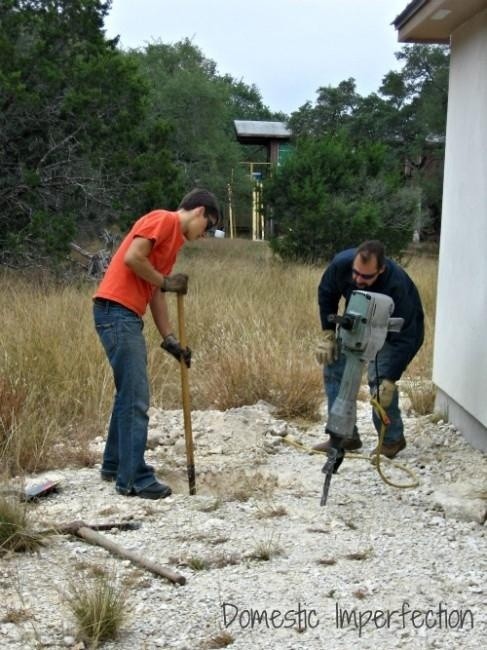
[369,434,406,460]
[115,480,172,499]
[100,464,157,482]
[310,426,363,453]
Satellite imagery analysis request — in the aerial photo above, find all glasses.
[204,215,215,231]
[352,269,380,280]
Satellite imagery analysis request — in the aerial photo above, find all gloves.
[315,329,338,367]
[160,333,192,368]
[370,379,396,410]
[160,272,189,296]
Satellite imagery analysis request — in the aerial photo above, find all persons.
[311,237,426,462]
[89,186,219,504]
